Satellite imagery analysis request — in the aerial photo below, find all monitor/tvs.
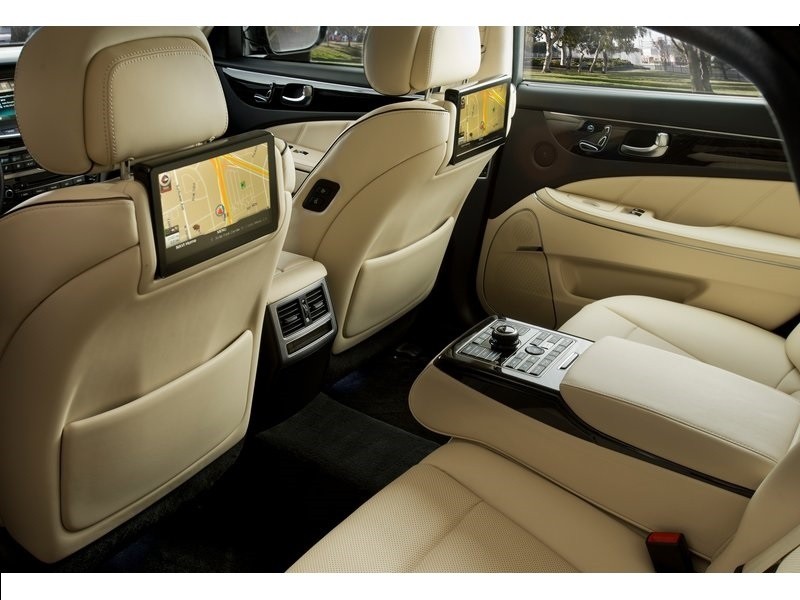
[444,73,511,163]
[138,128,279,271]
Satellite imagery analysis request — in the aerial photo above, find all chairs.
[280,25,516,362]
[279,440,800,572]
[1,26,292,567]
[556,291,800,397]
